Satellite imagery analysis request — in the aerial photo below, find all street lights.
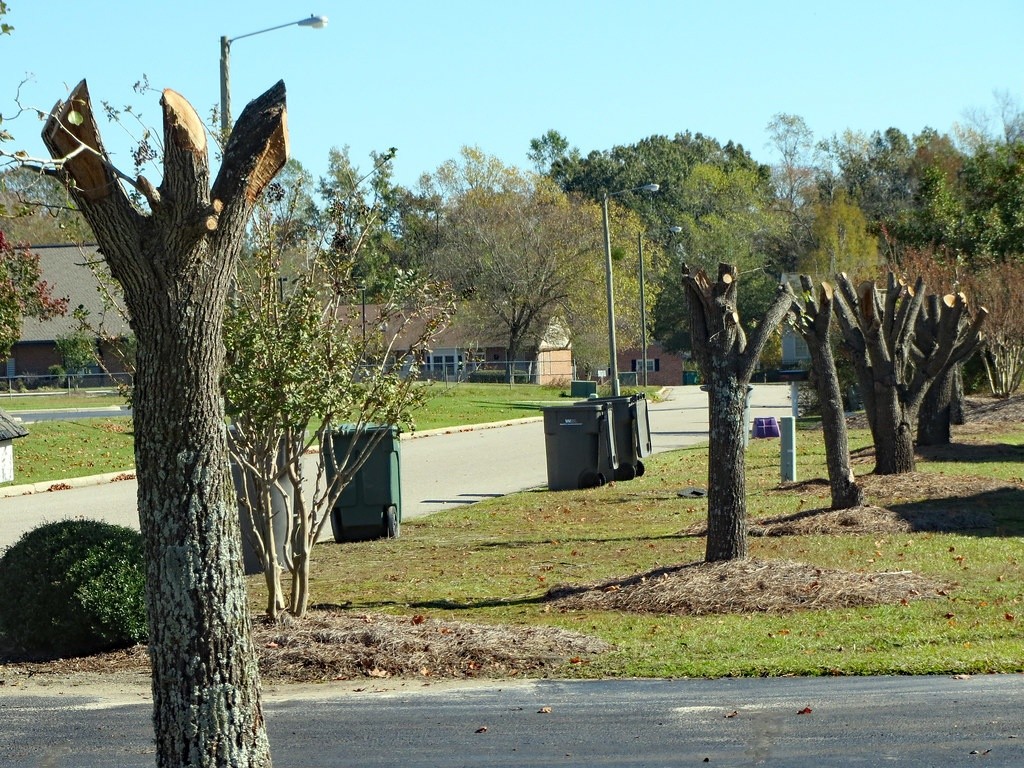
[637,224,684,388]
[218,14,330,323]
[600,182,659,396]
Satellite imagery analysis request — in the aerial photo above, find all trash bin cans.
[588,392,652,481]
[539,401,620,491]
[682,371,697,385]
[227,424,301,576]
[323,422,402,544]
